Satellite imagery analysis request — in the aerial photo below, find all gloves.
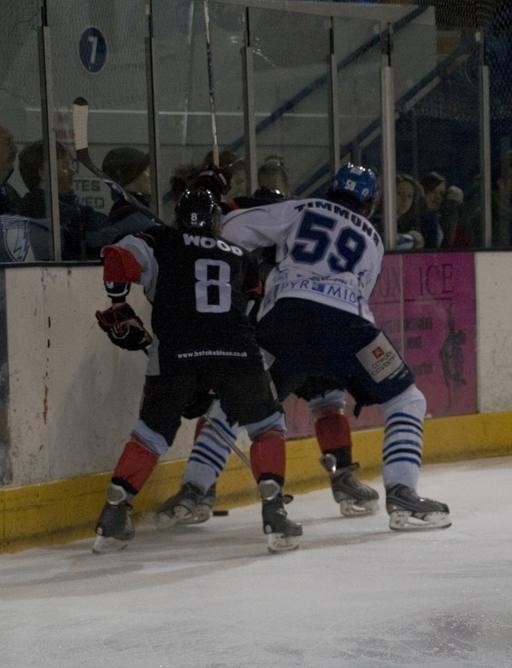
[96,303,153,350]
[181,397,212,418]
[195,164,231,202]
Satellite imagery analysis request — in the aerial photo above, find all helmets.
[174,186,222,240]
[326,162,383,219]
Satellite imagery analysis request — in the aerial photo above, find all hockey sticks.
[68,93,171,229]
[143,345,294,505]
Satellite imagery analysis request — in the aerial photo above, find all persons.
[1,128,511,262]
[152,187,381,527]
[155,161,453,531]
[93,186,302,554]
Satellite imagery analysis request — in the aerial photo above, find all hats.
[102,147,151,187]
